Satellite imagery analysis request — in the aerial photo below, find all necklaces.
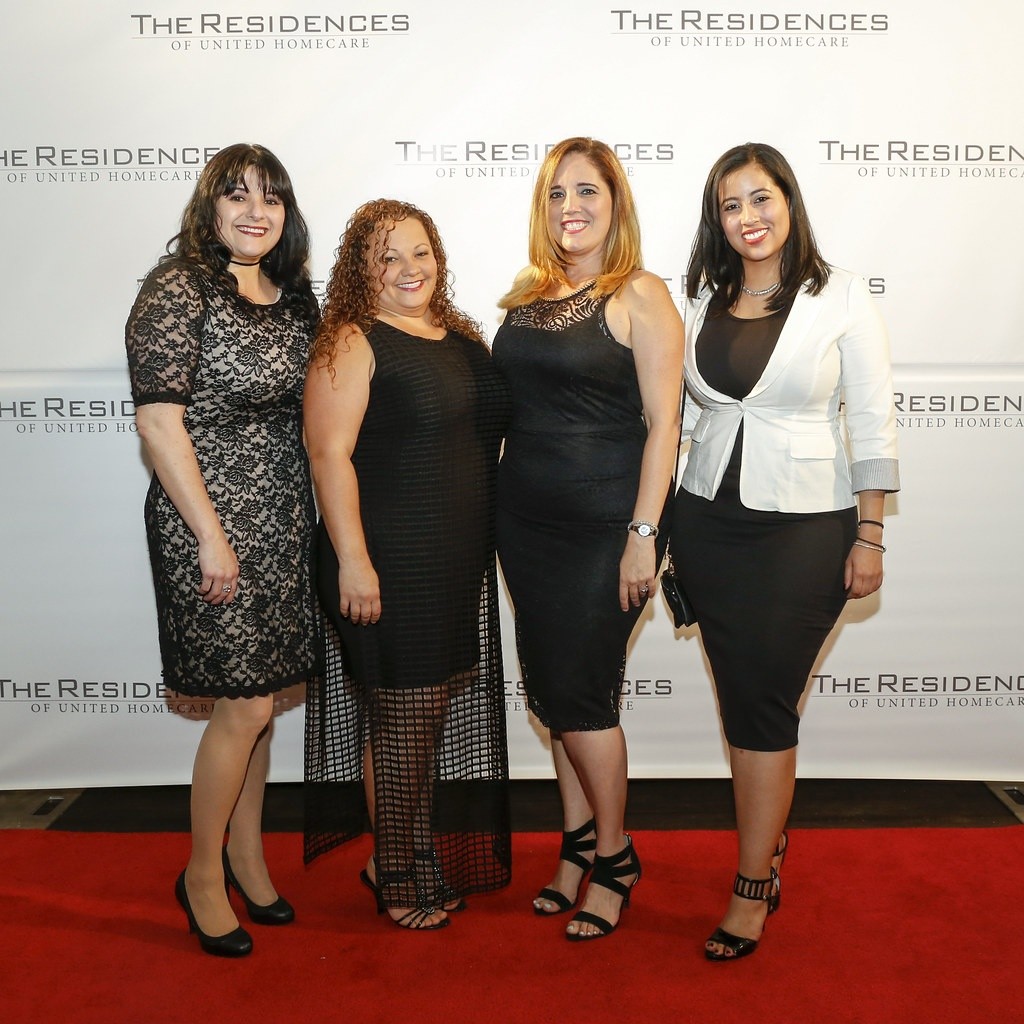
[741,284,778,295]
[229,260,261,266]
[538,279,598,301]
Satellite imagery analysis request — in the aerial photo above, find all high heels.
[565,832,641,941]
[771,831,788,868]
[175,866,253,959]
[222,845,295,926]
[360,850,467,930]
[705,866,781,960]
[533,815,598,916]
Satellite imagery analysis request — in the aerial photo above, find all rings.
[640,585,649,592]
[222,586,231,593]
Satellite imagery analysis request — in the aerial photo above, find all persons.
[668,142,899,962]
[125,143,329,958]
[301,197,513,930]
[489,137,686,942]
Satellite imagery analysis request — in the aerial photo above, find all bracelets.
[628,520,659,537]
[858,520,884,530]
[853,537,886,554]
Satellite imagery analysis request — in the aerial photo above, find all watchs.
[629,525,656,538]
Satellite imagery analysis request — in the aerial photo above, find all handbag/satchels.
[660,569,697,628]
